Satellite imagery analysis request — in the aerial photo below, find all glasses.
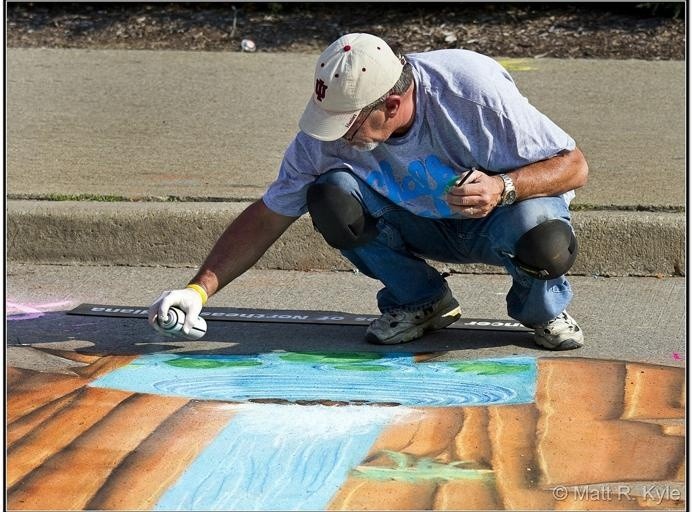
[342,105,378,143]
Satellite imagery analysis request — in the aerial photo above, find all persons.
[148,33,589,351]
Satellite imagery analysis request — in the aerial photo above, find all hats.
[298,33,404,142]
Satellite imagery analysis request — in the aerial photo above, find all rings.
[470,207,473,215]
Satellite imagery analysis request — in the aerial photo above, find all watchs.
[497,174,517,208]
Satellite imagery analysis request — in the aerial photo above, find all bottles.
[157,307,207,341]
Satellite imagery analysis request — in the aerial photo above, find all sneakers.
[533,309,584,350]
[364,272,461,345]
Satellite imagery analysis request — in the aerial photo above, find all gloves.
[148,284,207,335]
